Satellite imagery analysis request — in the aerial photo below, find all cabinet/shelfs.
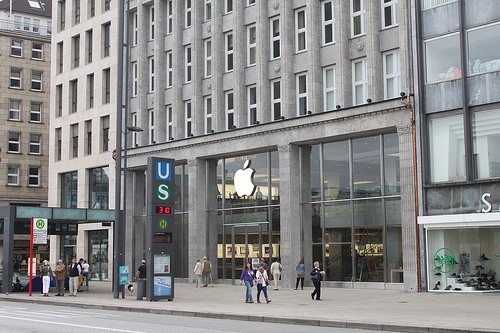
[447,259,500,290]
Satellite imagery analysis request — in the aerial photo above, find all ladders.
[351,225,378,282]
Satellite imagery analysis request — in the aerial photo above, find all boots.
[257,296,261,303]
[265,296,272,303]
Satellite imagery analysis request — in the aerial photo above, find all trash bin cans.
[137,278,147,301]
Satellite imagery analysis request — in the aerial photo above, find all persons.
[39,258,90,297]
[192,256,213,288]
[12,259,22,270]
[309,261,325,301]
[294,258,305,290]
[270,258,283,290]
[240,258,271,304]
[136,260,146,278]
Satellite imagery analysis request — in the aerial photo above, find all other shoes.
[250,300,254,303]
[246,300,249,302]
[433,253,500,291]
[55,293,61,296]
[316,298,320,300]
[73,294,76,297]
[311,293,314,299]
[69,294,72,296]
[44,294,48,296]
[61,294,64,296]
[294,288,298,290]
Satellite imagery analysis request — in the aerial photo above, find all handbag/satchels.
[311,273,318,280]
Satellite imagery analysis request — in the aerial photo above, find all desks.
[390,269,403,282]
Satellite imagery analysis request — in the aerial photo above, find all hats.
[142,260,145,263]
